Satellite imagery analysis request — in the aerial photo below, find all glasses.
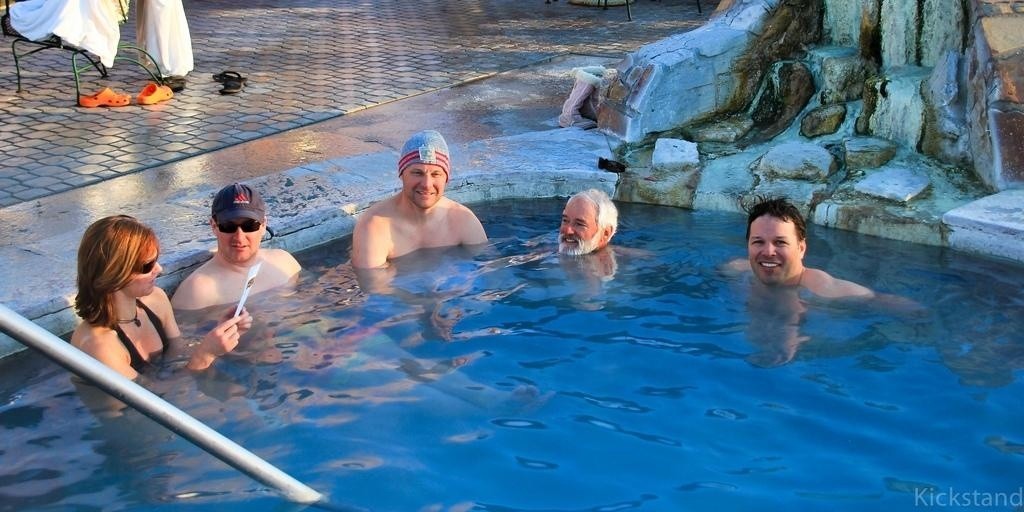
[216,219,261,233]
[137,255,159,274]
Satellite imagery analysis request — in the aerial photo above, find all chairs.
[1,1,163,105]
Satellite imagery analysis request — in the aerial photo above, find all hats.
[211,182,266,224]
[398,130,449,183]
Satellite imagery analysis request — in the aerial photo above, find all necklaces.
[116,310,141,327]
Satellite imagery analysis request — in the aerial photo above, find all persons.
[558,189,654,258]
[171,183,557,420]
[726,198,926,318]
[351,130,489,269]
[71,214,253,380]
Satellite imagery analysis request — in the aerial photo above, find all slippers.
[78,87,132,108]
[135,82,174,105]
[212,70,248,95]
